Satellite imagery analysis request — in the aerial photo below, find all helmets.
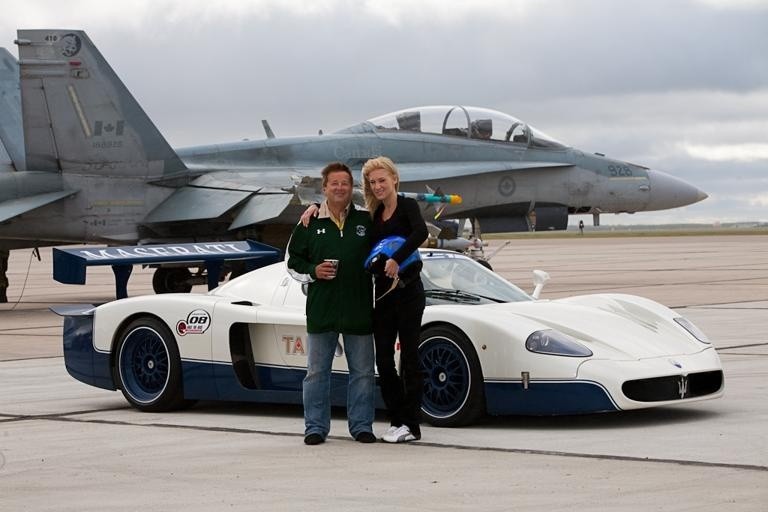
[364,236,423,289]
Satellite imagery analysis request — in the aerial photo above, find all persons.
[298,157,429,444]
[285,164,379,445]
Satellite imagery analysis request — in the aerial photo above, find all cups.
[323,259,339,279]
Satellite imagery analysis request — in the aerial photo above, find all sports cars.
[43,238,728,428]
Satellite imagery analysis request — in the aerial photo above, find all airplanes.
[0,28,710,297]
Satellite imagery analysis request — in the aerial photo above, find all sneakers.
[379,426,397,440]
[358,432,376,442]
[381,425,421,443]
[305,434,321,445]
